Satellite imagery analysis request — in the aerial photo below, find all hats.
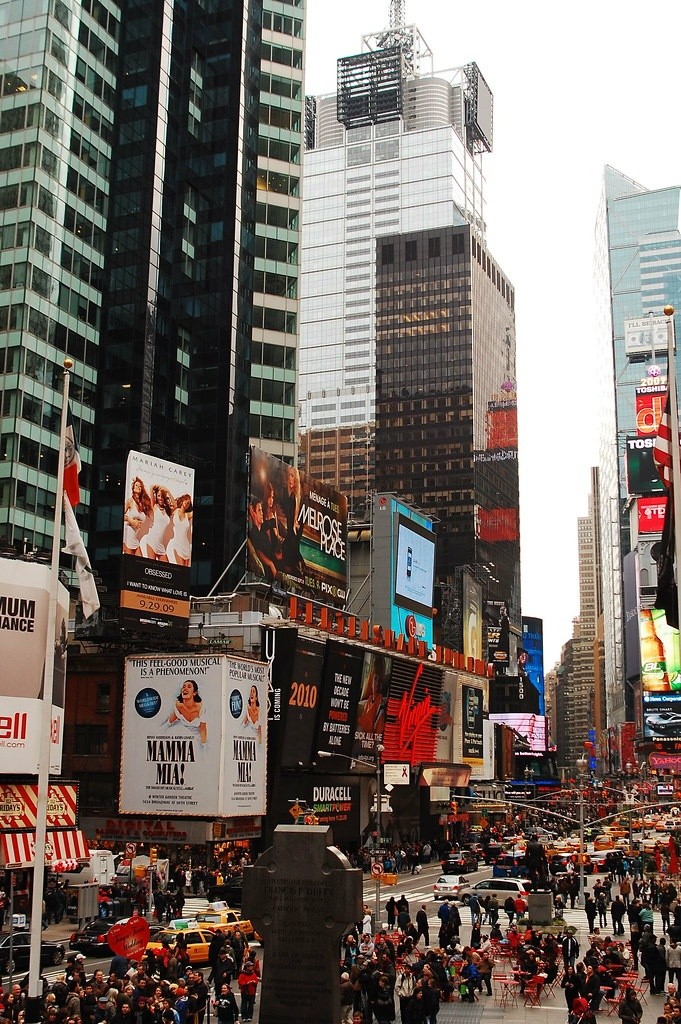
[626,988,636,999]
[599,892,605,897]
[341,972,349,980]
[218,950,228,955]
[75,954,87,960]
[169,983,178,991]
[245,961,254,966]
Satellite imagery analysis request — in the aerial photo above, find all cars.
[433,873,470,902]
[441,812,681,876]
[68,915,165,958]
[0,930,66,975]
[140,917,227,967]
[645,711,681,731]
[193,901,254,940]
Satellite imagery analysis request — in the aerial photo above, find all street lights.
[317,749,384,936]
[522,766,529,829]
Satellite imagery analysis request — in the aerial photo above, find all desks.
[593,986,613,1014]
[616,977,637,999]
[509,971,531,996]
[499,979,519,1008]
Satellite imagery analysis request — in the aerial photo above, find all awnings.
[0,830,90,872]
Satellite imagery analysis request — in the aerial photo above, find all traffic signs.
[361,835,393,856]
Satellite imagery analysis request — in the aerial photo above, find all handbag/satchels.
[450,965,455,975]
[460,984,467,995]
[394,905,398,916]
[477,901,486,914]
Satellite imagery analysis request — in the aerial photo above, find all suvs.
[457,877,535,910]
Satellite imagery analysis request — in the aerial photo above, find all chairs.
[635,979,651,1004]
[524,976,545,1008]
[606,985,628,1018]
[486,935,639,986]
[340,932,480,1002]
[493,974,508,1005]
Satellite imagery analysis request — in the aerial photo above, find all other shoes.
[486,993,492,997]
[425,946,431,949]
[479,987,483,992]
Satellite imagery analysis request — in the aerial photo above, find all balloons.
[50,858,78,872]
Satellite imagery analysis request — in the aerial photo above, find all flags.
[63,402,100,619]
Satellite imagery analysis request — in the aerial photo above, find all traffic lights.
[625,763,635,870]
[450,801,457,818]
[150,847,157,864]
[575,753,590,910]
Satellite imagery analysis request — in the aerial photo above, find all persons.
[358,656,387,737]
[0,874,69,931]
[249,465,312,590]
[499,607,511,634]
[357,768,681,908]
[0,893,681,1024]
[123,477,194,567]
[159,679,264,755]
[517,647,530,680]
[515,715,536,751]
[97,842,254,896]
